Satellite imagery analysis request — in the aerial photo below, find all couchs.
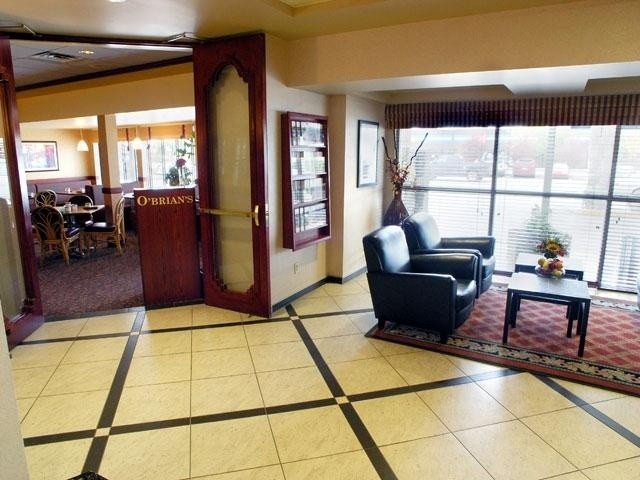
[84,180,139,214]
[26,176,92,207]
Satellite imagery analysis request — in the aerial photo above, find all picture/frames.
[356,118,380,188]
[21,140,59,171]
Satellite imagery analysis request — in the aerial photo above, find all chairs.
[80,197,127,257]
[31,206,84,270]
[35,189,57,208]
[400,210,496,299]
[66,195,93,255]
[362,224,478,344]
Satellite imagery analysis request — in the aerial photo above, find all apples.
[538,257,545,264]
[548,262,557,271]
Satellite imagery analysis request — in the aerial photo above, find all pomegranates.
[556,261,563,270]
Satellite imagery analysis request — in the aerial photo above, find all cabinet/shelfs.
[279,111,332,251]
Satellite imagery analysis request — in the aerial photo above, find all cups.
[80,187,85,191]
[84,203,92,207]
[62,201,78,211]
[66,188,71,192]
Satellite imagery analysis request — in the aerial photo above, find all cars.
[417,148,537,184]
[552,160,569,180]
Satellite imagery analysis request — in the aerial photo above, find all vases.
[382,189,409,226]
[535,264,554,277]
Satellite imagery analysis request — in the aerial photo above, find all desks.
[50,204,106,256]
[133,188,201,307]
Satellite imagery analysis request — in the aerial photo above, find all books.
[291,120,328,233]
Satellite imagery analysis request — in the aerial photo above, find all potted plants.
[164,167,179,186]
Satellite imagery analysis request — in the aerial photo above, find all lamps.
[131,126,144,149]
[77,128,88,152]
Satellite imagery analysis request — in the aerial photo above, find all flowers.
[176,158,191,185]
[534,233,569,278]
[381,128,428,192]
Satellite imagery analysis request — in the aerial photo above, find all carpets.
[364,281,640,396]
